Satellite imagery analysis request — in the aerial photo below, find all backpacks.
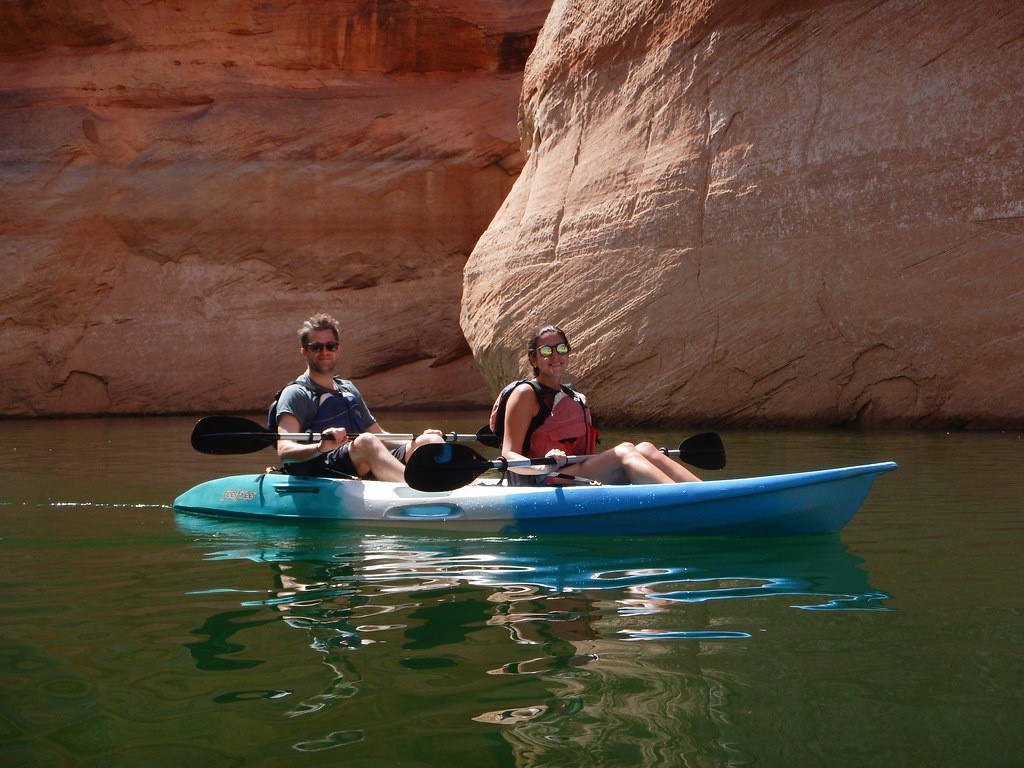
[268,379,320,452]
[489,377,584,446]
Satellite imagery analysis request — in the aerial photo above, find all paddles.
[191,415,504,455]
[403,432,727,494]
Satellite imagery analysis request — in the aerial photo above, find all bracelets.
[317,443,326,453]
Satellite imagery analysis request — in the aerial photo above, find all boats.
[172,461,898,537]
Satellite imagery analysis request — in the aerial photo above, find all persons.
[274,313,447,482]
[501,324,702,486]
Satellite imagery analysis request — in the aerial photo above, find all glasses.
[532,342,571,357]
[308,341,339,353]
